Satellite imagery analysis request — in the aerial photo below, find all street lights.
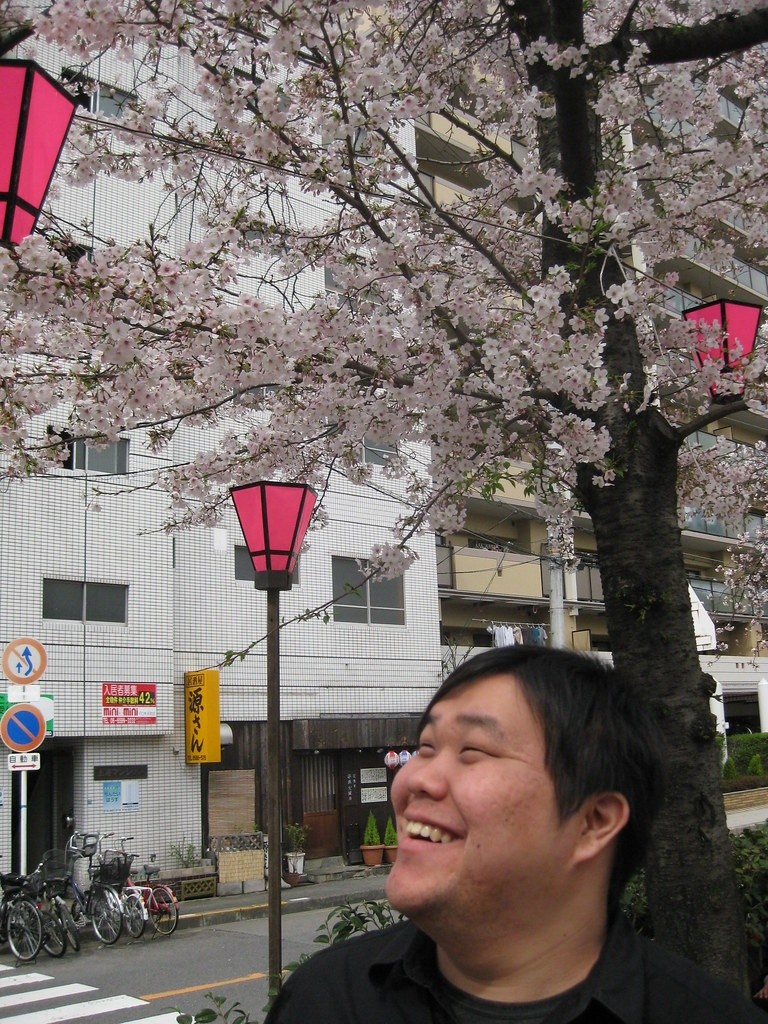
[228,480,319,1014]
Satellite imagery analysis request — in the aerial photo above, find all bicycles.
[0,830,181,968]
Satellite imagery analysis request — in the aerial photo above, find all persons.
[263,642,768,1024]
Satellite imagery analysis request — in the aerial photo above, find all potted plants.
[280,843,304,886]
[160,842,216,880]
[383,816,402,864]
[359,810,384,866]
[284,824,308,875]
